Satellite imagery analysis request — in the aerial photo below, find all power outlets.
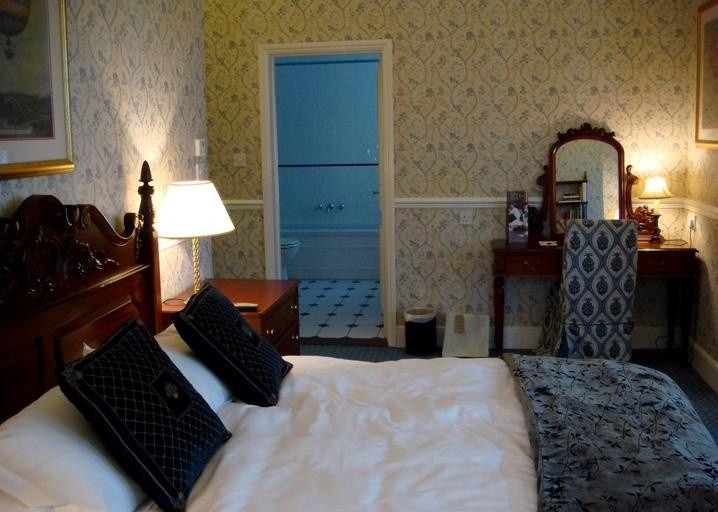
[460,212,474,226]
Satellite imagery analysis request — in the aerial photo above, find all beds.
[2,161,718,511]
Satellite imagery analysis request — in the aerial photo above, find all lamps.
[153,181,235,303]
[639,175,675,244]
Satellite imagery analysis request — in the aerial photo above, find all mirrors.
[547,122,626,240]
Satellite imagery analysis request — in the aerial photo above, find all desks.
[491,238,698,369]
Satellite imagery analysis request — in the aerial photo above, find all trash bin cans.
[403,307,437,356]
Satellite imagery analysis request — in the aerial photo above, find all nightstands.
[162,279,300,354]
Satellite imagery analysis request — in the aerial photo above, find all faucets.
[328,203,335,216]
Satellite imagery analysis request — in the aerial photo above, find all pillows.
[59,318,232,512]
[174,281,293,408]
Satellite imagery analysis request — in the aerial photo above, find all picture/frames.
[1,1,77,179]
[694,0,718,148]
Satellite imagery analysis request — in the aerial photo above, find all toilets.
[281,239,304,280]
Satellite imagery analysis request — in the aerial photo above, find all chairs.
[534,219,638,361]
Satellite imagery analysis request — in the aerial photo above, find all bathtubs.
[280,223,380,279]
